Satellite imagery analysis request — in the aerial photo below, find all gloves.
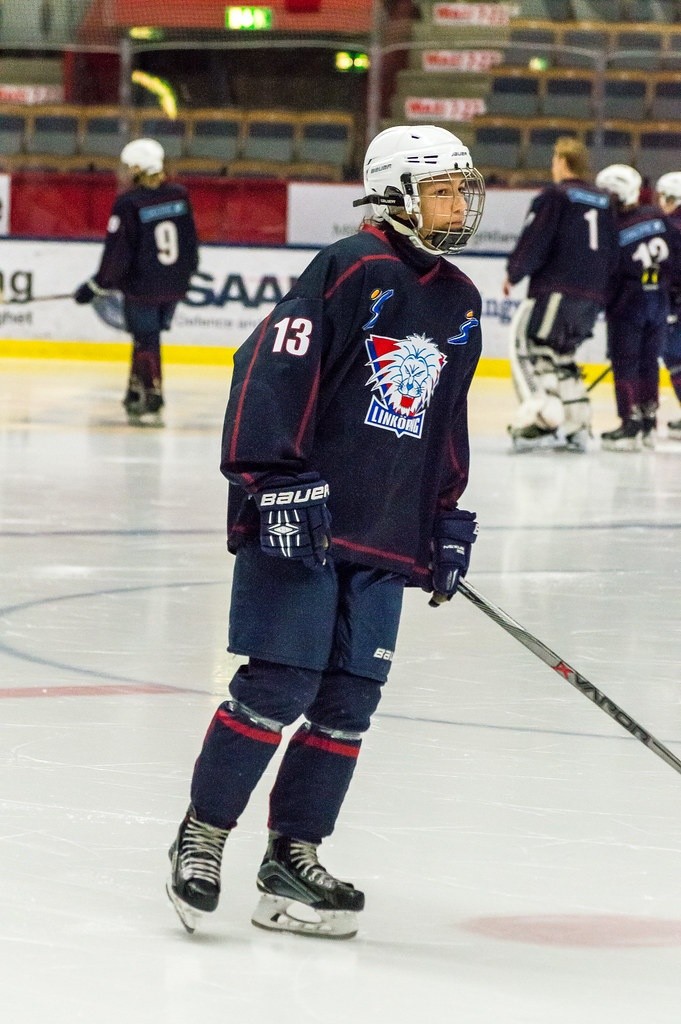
[428,508,481,608]
[252,471,333,572]
[72,283,95,304]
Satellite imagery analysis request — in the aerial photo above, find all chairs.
[0,104,355,183]
[388,0,680,191]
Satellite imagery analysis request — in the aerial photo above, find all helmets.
[595,164,642,205]
[119,137,164,177]
[352,124,485,256]
[655,172,681,197]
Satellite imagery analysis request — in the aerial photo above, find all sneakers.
[667,421,681,440]
[600,414,643,451]
[161,804,238,934]
[251,829,365,940]
[506,424,558,453]
[121,389,165,427]
[640,417,657,450]
[553,432,588,451]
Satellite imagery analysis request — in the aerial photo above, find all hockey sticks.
[457,573,680,772]
[585,364,613,393]
[1,292,72,307]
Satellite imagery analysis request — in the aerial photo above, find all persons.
[595,164,672,451]
[656,172,681,438]
[73,138,199,426]
[503,136,620,453]
[164,125,487,940]
